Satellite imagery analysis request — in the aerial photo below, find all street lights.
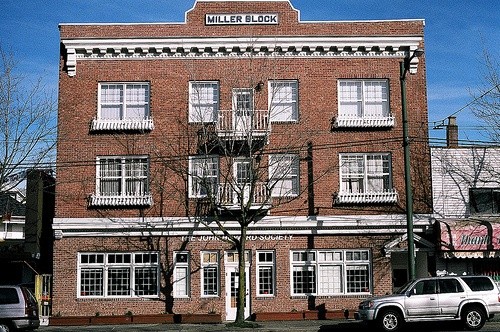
[395,45,425,287]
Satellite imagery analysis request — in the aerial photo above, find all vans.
[0,284,40,332]
[355,273,500,330]
